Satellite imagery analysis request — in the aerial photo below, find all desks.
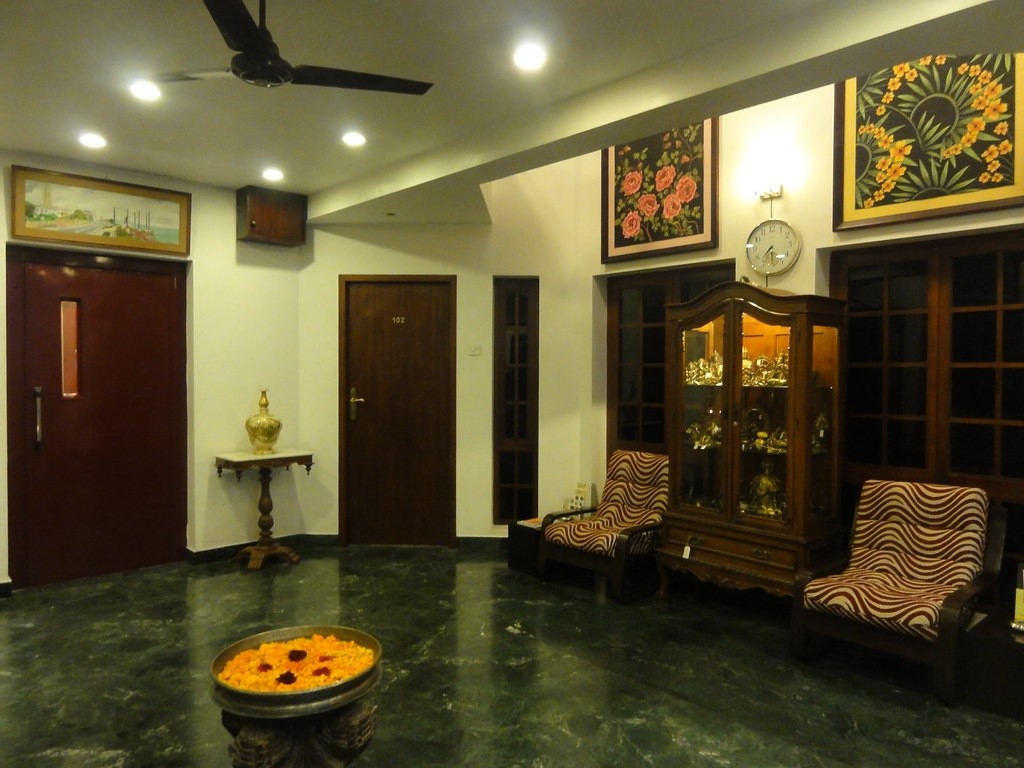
[214,451,314,570]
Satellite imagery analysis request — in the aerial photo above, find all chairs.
[798,478,1010,691]
[537,450,671,602]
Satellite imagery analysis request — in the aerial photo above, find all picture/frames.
[600,112,719,265]
[9,163,192,257]
[830,49,1023,232]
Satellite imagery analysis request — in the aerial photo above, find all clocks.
[744,181,802,276]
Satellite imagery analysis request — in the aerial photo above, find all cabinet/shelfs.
[661,283,846,600]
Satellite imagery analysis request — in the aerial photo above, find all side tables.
[507,513,572,574]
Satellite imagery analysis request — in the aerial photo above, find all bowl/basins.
[208,625,383,705]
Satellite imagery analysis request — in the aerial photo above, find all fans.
[155,0,433,97]
